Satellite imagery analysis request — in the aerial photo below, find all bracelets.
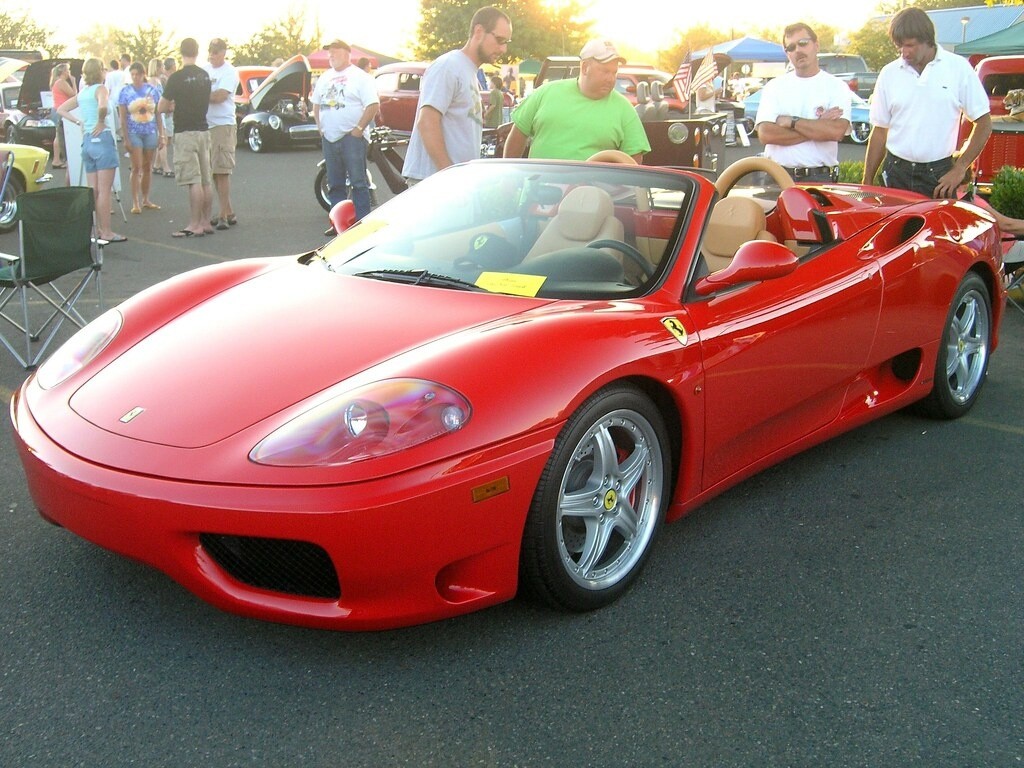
[159,135,165,138]
[356,125,363,132]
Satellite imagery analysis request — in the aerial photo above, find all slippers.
[205,227,215,235]
[131,207,142,214]
[98,232,127,242]
[53,162,67,169]
[143,202,161,209]
[172,228,204,237]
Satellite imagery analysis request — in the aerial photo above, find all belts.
[784,166,832,177]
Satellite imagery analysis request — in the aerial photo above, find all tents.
[686,36,789,121]
[305,41,380,71]
[952,20,1024,57]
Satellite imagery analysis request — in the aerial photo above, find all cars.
[366,60,516,132]
[0,49,96,146]
[958,53,1024,195]
[231,54,323,153]
[0,143,53,234]
[738,88,872,145]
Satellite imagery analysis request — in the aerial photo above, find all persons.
[483,76,504,136]
[754,21,854,184]
[55,58,129,243]
[730,71,746,98]
[205,37,240,229]
[500,36,652,191]
[157,37,215,239]
[118,62,167,214]
[48,53,177,177]
[519,77,525,97]
[356,57,383,162]
[310,38,381,236]
[862,7,993,240]
[401,6,513,189]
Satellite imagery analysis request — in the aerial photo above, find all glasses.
[785,39,814,52]
[482,25,512,45]
[209,50,220,54]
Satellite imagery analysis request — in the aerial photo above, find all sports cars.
[9,149,1010,633]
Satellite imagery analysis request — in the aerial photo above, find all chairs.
[0,186,111,373]
[1002,234,1024,312]
[520,186,622,266]
[701,197,780,273]
[651,80,670,120]
[633,81,654,122]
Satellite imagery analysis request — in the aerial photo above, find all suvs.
[527,56,744,136]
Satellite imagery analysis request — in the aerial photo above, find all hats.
[208,38,226,52]
[580,39,626,65]
[323,40,351,53]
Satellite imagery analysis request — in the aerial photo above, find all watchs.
[791,116,799,128]
[76,120,80,126]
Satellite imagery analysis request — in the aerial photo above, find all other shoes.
[325,226,337,236]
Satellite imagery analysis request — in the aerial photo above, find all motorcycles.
[314,81,730,212]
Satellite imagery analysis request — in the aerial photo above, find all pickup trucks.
[786,54,879,100]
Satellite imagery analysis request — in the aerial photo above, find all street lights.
[960,16,969,43]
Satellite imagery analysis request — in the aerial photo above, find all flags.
[690,45,719,97]
[671,45,693,102]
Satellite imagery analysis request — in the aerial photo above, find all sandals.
[211,212,237,225]
[152,166,163,175]
[162,170,175,178]
[217,217,229,229]
